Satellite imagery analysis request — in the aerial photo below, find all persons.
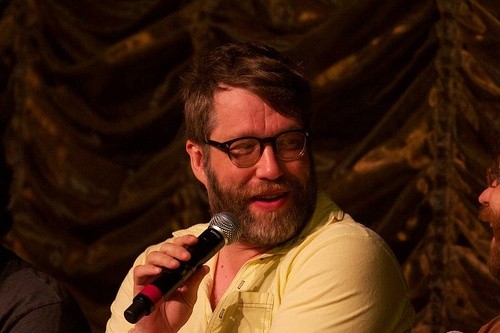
[105,43,414,332]
[477,157,500,333]
[0,157,94,333]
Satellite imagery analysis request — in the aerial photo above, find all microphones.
[124,211,243,324]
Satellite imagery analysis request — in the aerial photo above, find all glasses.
[486,167,500,188]
[204,128,311,168]
